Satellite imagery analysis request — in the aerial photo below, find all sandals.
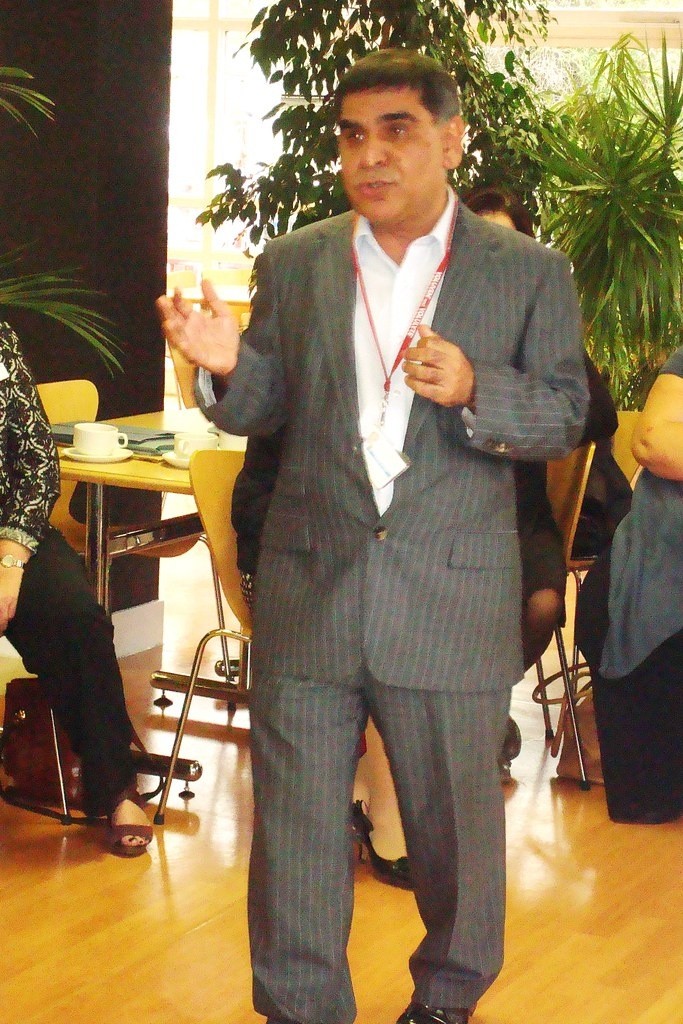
[105,788,153,854]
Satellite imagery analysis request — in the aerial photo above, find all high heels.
[352,799,413,888]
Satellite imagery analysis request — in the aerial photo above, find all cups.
[219,429,248,452]
[72,423,129,455]
[173,431,219,460]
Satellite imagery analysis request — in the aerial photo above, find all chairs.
[535,440,599,791]
[155,449,251,824]
[572,409,647,717]
[168,297,254,685]
[39,379,105,827]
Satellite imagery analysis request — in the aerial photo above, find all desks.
[54,409,254,799]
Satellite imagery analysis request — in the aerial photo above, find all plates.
[162,452,190,469]
[63,447,133,463]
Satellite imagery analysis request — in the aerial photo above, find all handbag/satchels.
[531,662,604,785]
[0,678,163,825]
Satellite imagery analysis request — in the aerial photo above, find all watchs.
[1,555,26,569]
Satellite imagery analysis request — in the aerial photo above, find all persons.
[156,47,588,1024]
[1,320,153,856]
[229,193,683,884]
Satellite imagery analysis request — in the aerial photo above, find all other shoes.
[396,1000,469,1024]
[502,714,522,760]
[499,757,511,782]
